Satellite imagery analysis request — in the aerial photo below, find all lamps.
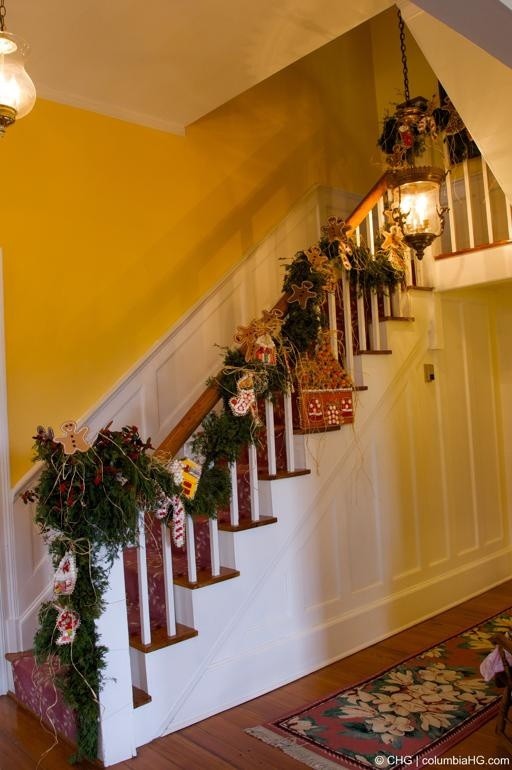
[384,11,452,260]
[0,0,38,139]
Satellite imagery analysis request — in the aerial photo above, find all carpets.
[243,606,512,769]
